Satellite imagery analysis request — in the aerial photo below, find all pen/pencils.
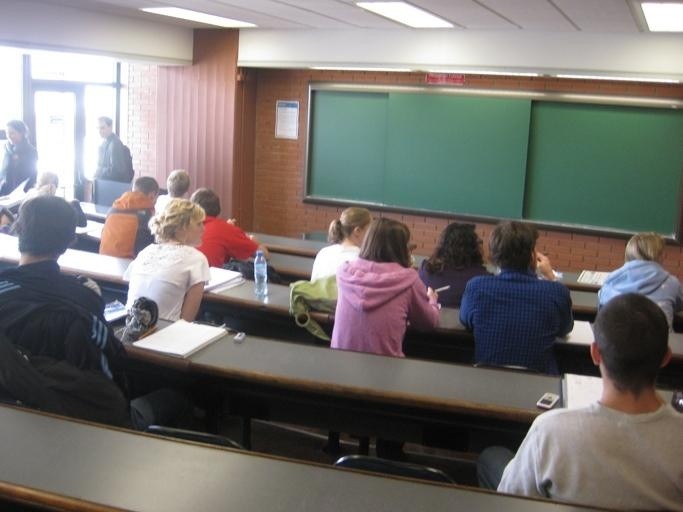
[138,325,159,340]
[434,285,450,292]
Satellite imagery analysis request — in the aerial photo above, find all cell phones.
[537,392,560,410]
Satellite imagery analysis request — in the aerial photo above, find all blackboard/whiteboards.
[302,80,683,247]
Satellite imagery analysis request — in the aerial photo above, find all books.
[133,319,227,360]
[577,270,610,286]
[202,266,246,295]
[105,301,127,324]
[556,321,594,346]
[562,373,603,412]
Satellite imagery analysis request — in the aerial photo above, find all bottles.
[254,250,267,296]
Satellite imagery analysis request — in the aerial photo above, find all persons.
[189,188,270,269]
[153,170,191,244]
[476,294,682,512]
[328,217,443,358]
[0,120,38,196]
[0,195,164,399]
[597,231,682,334]
[98,175,160,259]
[123,196,211,322]
[0,172,87,247]
[419,223,498,308]
[92,117,134,181]
[459,221,574,374]
[311,207,374,282]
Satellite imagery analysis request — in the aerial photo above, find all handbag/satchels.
[288,274,337,342]
